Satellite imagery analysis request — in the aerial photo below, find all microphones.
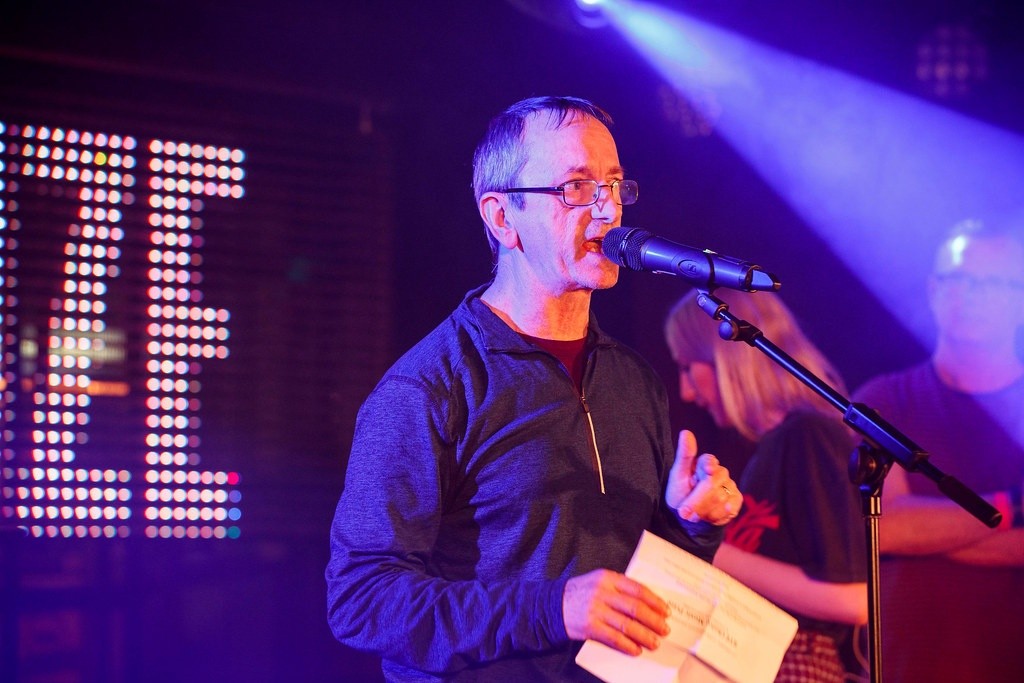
[602,227,782,292]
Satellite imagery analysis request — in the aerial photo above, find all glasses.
[933,271,1023,300]
[496,178,640,208]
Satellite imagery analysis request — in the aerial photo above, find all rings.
[721,484,734,497]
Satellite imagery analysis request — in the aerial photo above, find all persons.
[846,218,1020,681]
[669,286,876,683]
[326,93,744,683]
